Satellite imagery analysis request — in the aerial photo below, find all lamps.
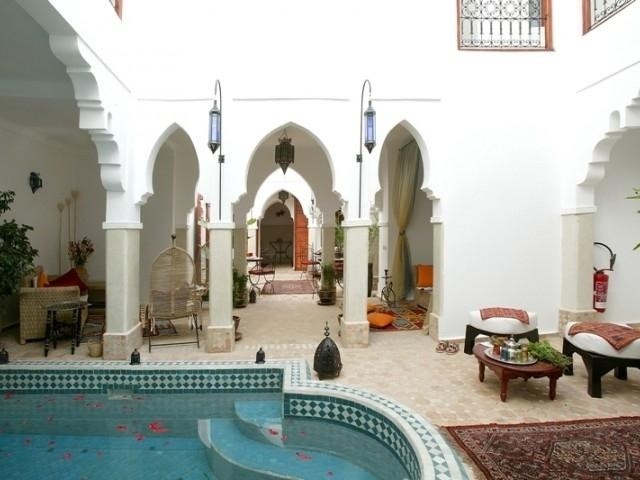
[358,79,377,218]
[274,128,294,175]
[209,79,225,220]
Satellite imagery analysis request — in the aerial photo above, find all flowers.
[68,233,94,266]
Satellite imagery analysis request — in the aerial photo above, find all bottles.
[490,336,531,362]
[31,276,37,289]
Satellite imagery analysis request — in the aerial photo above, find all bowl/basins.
[89,343,103,357]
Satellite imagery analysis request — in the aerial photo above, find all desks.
[473,344,563,402]
[41,302,92,356]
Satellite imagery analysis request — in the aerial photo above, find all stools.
[463,308,539,354]
[563,322,640,398]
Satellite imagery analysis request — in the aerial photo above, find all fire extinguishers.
[593,267,616,313]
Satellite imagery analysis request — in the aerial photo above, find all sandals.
[436,341,447,352]
[447,343,458,352]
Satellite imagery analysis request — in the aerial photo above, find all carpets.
[368,299,427,332]
[439,416,640,480]
[261,279,320,295]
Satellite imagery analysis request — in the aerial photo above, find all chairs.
[147,246,202,352]
[300,242,318,280]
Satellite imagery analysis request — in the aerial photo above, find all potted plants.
[334,226,344,258]
[233,269,248,307]
[318,263,335,303]
[368,226,378,297]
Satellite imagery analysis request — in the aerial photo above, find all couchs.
[18,265,88,345]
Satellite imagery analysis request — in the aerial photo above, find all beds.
[414,264,433,310]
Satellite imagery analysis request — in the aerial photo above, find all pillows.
[44,268,89,293]
[367,312,393,327]
[38,271,49,287]
